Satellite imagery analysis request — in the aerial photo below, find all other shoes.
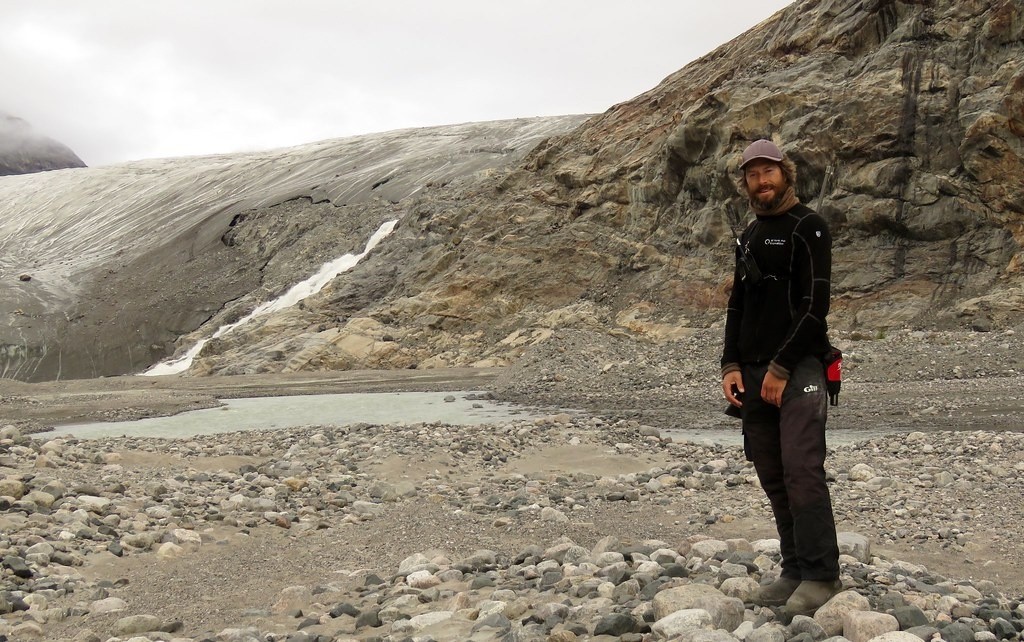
[748,577,802,606]
[786,578,843,617]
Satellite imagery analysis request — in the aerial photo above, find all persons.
[719,139,844,620]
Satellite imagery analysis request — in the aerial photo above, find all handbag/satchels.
[823,346,842,406]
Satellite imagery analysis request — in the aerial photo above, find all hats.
[739,139,782,170]
